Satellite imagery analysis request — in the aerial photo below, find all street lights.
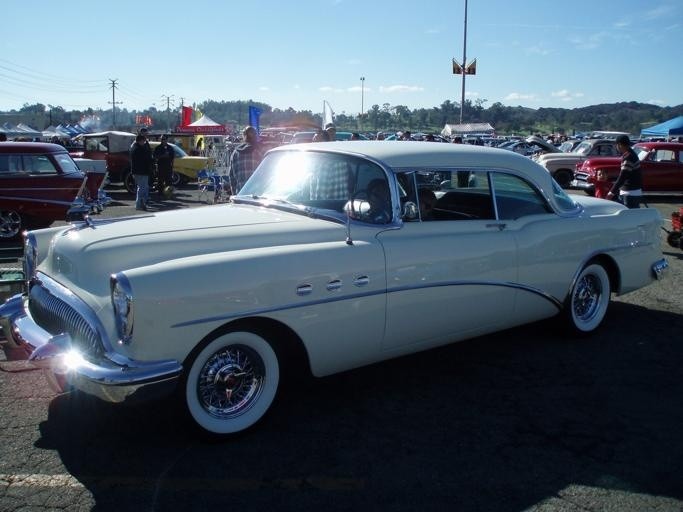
[360,74,365,114]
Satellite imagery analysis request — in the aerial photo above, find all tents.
[0,124,95,141]
[178,113,226,131]
[640,116,682,144]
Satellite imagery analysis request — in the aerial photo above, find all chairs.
[198,168,221,204]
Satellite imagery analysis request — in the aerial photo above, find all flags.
[180,107,192,128]
[194,109,202,121]
[248,105,262,138]
[322,101,333,130]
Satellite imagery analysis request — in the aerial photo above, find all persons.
[229,128,269,195]
[606,134,642,210]
[535,133,568,145]
[309,133,348,200]
[130,128,184,210]
[585,170,618,200]
[377,130,484,147]
[351,133,360,140]
[15,135,82,148]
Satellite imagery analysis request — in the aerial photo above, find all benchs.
[431,191,545,220]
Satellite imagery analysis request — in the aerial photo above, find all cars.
[0,128,683,245]
[0,139,670,443]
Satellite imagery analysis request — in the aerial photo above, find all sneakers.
[135,192,178,210]
